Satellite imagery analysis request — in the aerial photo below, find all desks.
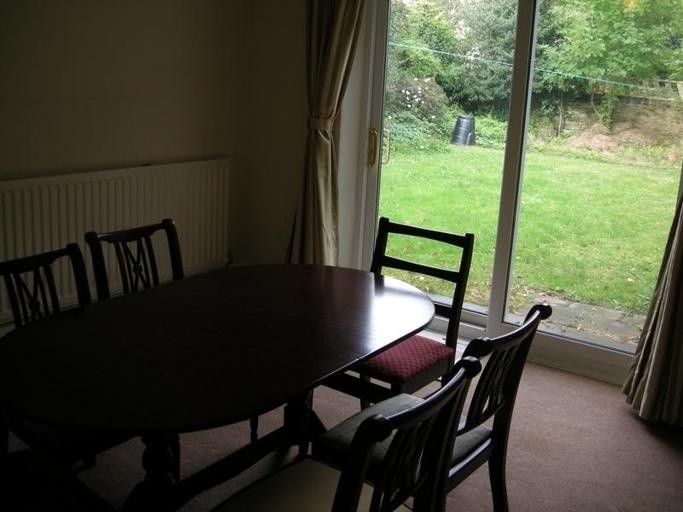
[2,266,437,511]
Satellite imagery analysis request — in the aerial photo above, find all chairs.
[214,356,482,512]
[307,217,475,412]
[3,242,92,325]
[86,218,186,299]
[317,300,551,511]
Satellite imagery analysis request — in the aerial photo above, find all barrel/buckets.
[452,115,477,145]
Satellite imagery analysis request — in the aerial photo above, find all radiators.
[0,156,231,325]
